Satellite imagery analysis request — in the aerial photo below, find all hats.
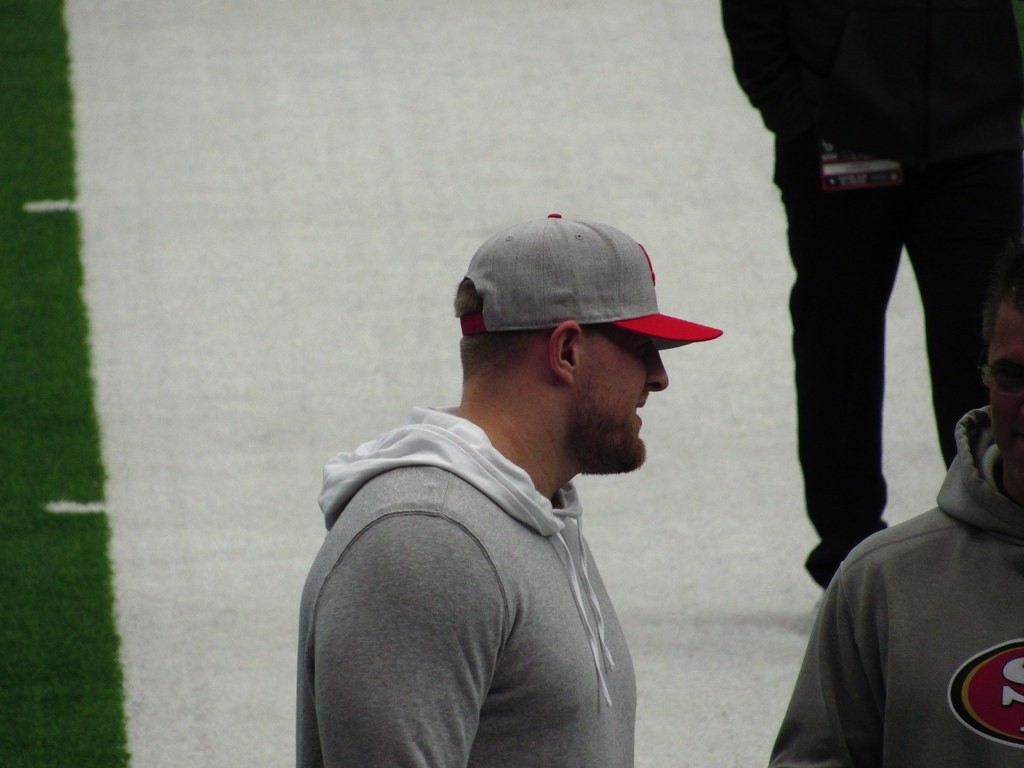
[460,213,723,351]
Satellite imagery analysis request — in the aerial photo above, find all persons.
[294,214,723,768]
[767,239,1024,768]
[721,0,1024,590]
